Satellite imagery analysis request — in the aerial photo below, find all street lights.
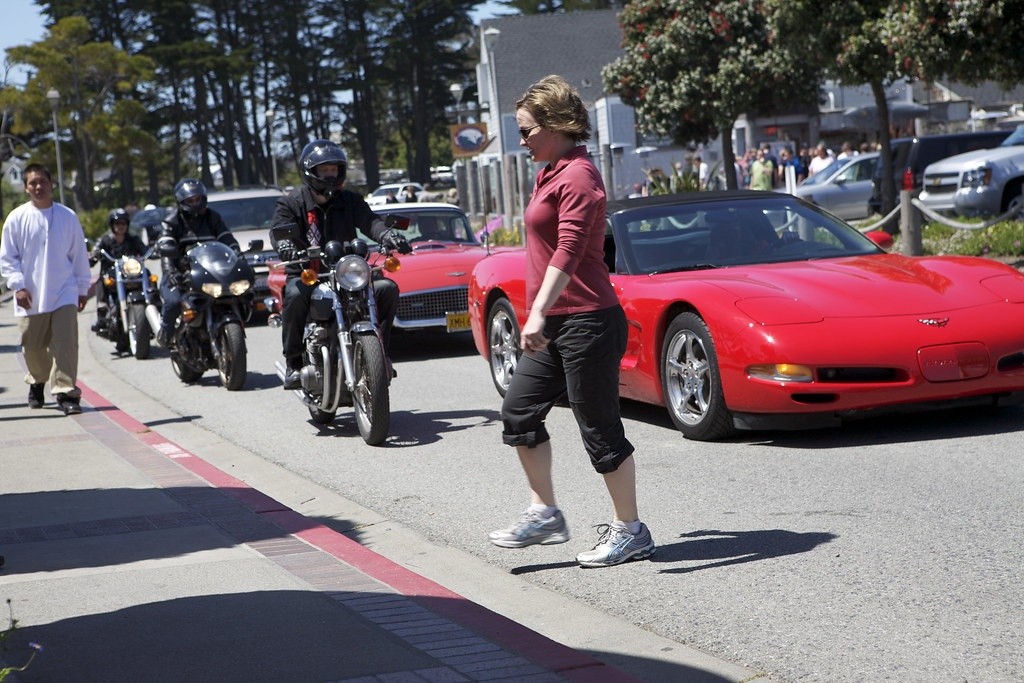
[483,25,505,155]
[47,88,65,205]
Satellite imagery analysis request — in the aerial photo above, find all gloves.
[379,230,413,255]
[277,239,298,269]
[170,272,185,285]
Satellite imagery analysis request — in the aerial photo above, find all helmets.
[297,141,346,194]
[173,179,207,215]
[109,208,130,234]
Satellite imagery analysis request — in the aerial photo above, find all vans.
[868,130,1014,231]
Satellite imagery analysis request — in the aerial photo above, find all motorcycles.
[85,237,172,359]
[146,218,263,391]
[263,213,413,445]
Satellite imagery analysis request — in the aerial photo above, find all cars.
[365,183,429,208]
[126,201,175,248]
[773,152,884,223]
[264,202,493,356]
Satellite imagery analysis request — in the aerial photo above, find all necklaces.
[30,200,54,246]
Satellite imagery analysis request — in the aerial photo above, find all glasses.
[519,124,542,139]
[114,221,125,225]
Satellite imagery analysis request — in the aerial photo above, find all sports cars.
[469,190,1024,442]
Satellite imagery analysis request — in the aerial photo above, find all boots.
[91,307,108,331]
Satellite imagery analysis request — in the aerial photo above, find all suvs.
[204,185,291,324]
[919,121,1024,224]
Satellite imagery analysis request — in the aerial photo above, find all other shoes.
[158,326,170,345]
[57,394,82,414]
[28,383,44,408]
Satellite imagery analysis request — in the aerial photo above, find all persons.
[269,141,413,390]
[489,74,657,568]
[624,141,885,199]
[156,178,256,348]
[0,163,91,415]
[386,184,460,205]
[89,208,163,333]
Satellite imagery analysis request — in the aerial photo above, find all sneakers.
[488,508,569,548]
[575,521,656,566]
[284,367,302,388]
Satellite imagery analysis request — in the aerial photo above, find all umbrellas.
[842,101,931,138]
